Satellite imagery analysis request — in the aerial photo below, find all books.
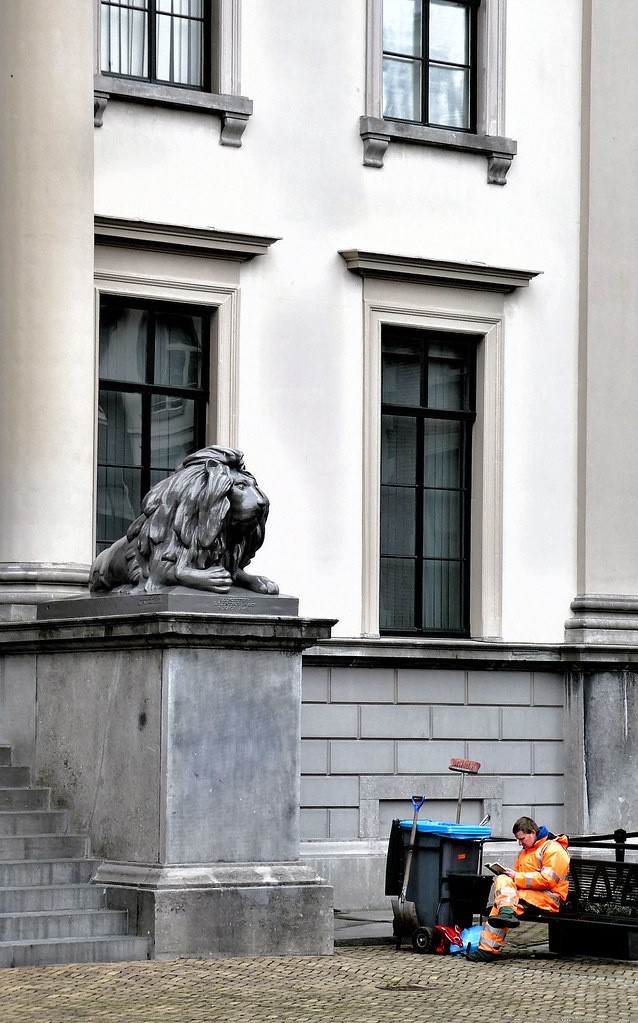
[484,862,506,876]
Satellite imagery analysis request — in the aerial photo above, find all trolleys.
[411,872,497,953]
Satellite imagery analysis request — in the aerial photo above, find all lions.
[88,445,280,595]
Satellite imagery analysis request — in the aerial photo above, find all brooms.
[448,757,482,824]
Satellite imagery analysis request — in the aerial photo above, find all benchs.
[512,840,638,934]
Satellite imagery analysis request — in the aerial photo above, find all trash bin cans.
[385,817,495,953]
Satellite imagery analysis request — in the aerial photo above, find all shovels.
[390,795,425,934]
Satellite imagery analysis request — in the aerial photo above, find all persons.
[466,816,571,961]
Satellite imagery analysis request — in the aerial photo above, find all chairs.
[435,872,495,928]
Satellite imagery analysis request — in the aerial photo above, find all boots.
[465,950,497,962]
[488,907,520,928]
